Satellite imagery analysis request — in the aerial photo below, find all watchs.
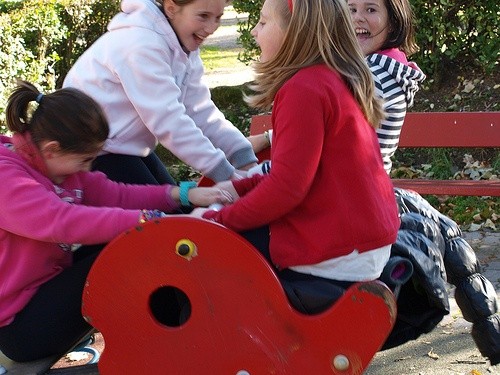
[179,181,198,207]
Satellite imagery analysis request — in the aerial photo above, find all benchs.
[250,112,500,201]
[0,325,97,375]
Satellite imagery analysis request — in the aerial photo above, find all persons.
[0,81,234,363]
[58,0,258,213]
[346,0,426,179]
[191,0,400,316]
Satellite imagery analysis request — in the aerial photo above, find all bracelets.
[264,129,272,145]
